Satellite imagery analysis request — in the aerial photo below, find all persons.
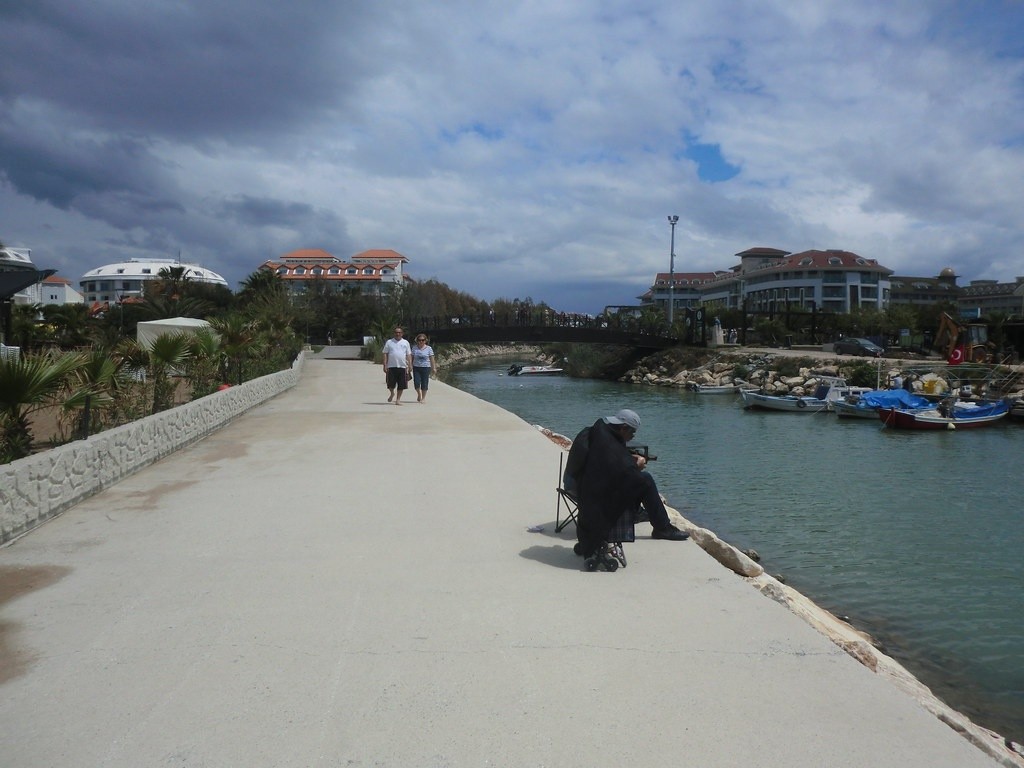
[326,329,336,346]
[488,304,607,330]
[892,373,914,394]
[723,328,739,344]
[382,328,411,405]
[410,334,437,404]
[563,409,690,539]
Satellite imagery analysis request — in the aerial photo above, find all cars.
[833,337,885,357]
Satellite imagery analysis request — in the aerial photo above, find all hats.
[605,409,641,428]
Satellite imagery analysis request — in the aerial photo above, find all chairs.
[555,451,580,535]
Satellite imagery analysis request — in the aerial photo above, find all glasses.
[418,339,425,342]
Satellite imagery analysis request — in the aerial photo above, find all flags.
[948,344,964,365]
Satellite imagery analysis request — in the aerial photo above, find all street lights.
[666,214,680,329]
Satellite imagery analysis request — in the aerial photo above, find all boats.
[877,393,1010,430]
[829,363,940,421]
[738,374,874,413]
[505,362,565,376]
[689,381,746,395]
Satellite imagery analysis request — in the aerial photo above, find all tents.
[134,315,212,357]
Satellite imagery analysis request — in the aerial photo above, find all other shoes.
[633,507,649,525]
[651,524,689,541]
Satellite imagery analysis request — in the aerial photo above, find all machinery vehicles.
[944,323,998,364]
